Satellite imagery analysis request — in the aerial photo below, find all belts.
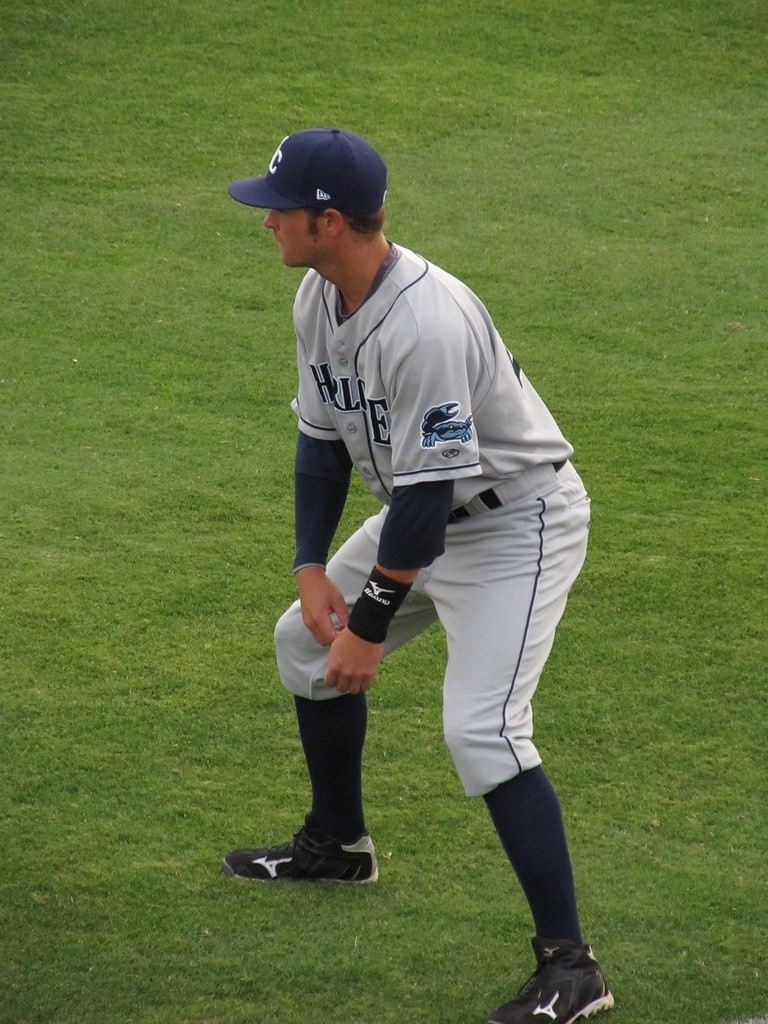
[447,459,567,525]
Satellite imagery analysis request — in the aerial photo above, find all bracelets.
[347,565,414,644]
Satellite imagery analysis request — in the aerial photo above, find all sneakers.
[222,813,379,885]
[488,936,614,1024]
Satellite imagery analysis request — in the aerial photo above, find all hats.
[228,127,388,213]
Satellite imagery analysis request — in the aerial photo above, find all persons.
[222,129,614,1024]
